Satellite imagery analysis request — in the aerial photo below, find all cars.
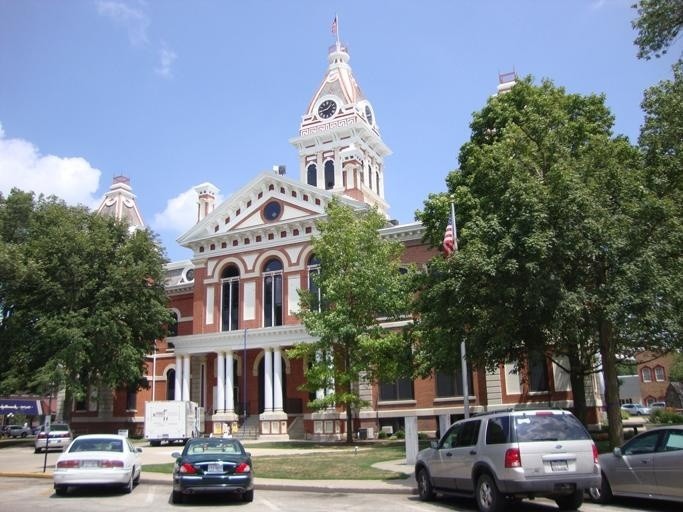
[32,425,44,435]
[171,437,255,505]
[34,423,73,455]
[52,434,144,496]
[584,423,682,505]
[651,402,666,411]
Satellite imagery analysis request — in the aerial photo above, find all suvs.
[1,424,30,439]
[620,404,650,417]
[414,404,603,512]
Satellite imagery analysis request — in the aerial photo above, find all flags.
[442,210,454,256]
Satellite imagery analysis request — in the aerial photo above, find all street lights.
[43,375,55,473]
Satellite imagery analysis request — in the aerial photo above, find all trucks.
[143,399,201,447]
